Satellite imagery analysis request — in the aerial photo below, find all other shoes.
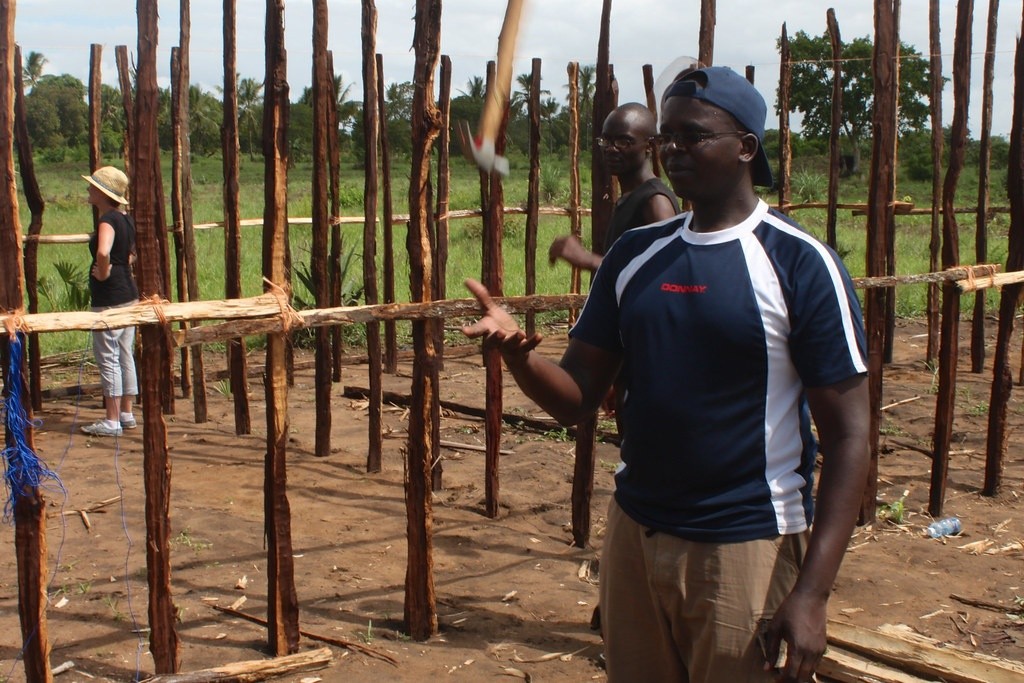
[81,420,122,436]
[120,420,137,428]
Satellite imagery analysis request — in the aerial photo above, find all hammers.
[453,0,524,178]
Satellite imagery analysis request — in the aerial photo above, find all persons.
[550,103,683,669]
[462,65,871,683]
[81,166,138,436]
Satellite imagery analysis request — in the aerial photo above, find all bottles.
[926,518,962,536]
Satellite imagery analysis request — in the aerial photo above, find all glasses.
[596,137,653,149]
[657,130,749,146]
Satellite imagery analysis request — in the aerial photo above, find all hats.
[664,66,772,187]
[82,166,128,205]
